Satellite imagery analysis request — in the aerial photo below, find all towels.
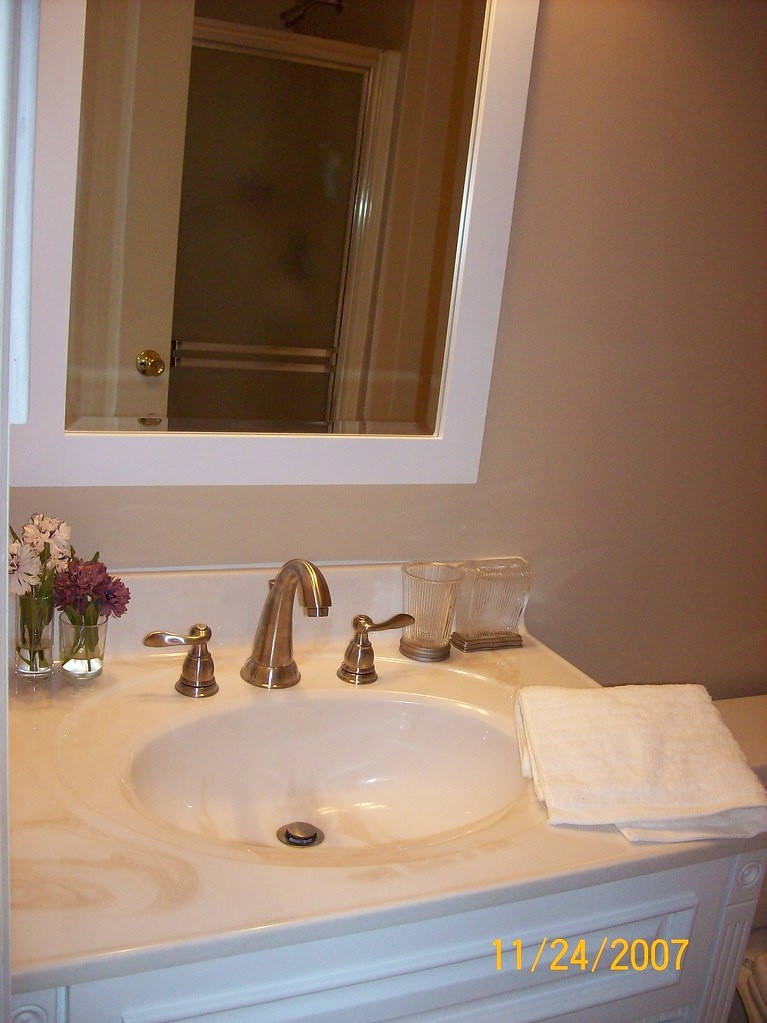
[516,683,766,841]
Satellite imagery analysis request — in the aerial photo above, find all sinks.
[52,657,549,871]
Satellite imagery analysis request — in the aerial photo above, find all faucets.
[242,558,330,690]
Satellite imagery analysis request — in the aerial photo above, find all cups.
[398,563,463,662]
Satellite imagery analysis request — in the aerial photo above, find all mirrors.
[10,0,540,487]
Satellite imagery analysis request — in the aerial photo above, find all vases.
[58,610,109,682]
[13,586,55,685]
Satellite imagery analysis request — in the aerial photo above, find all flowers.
[51,544,130,625]
[8,510,70,619]
